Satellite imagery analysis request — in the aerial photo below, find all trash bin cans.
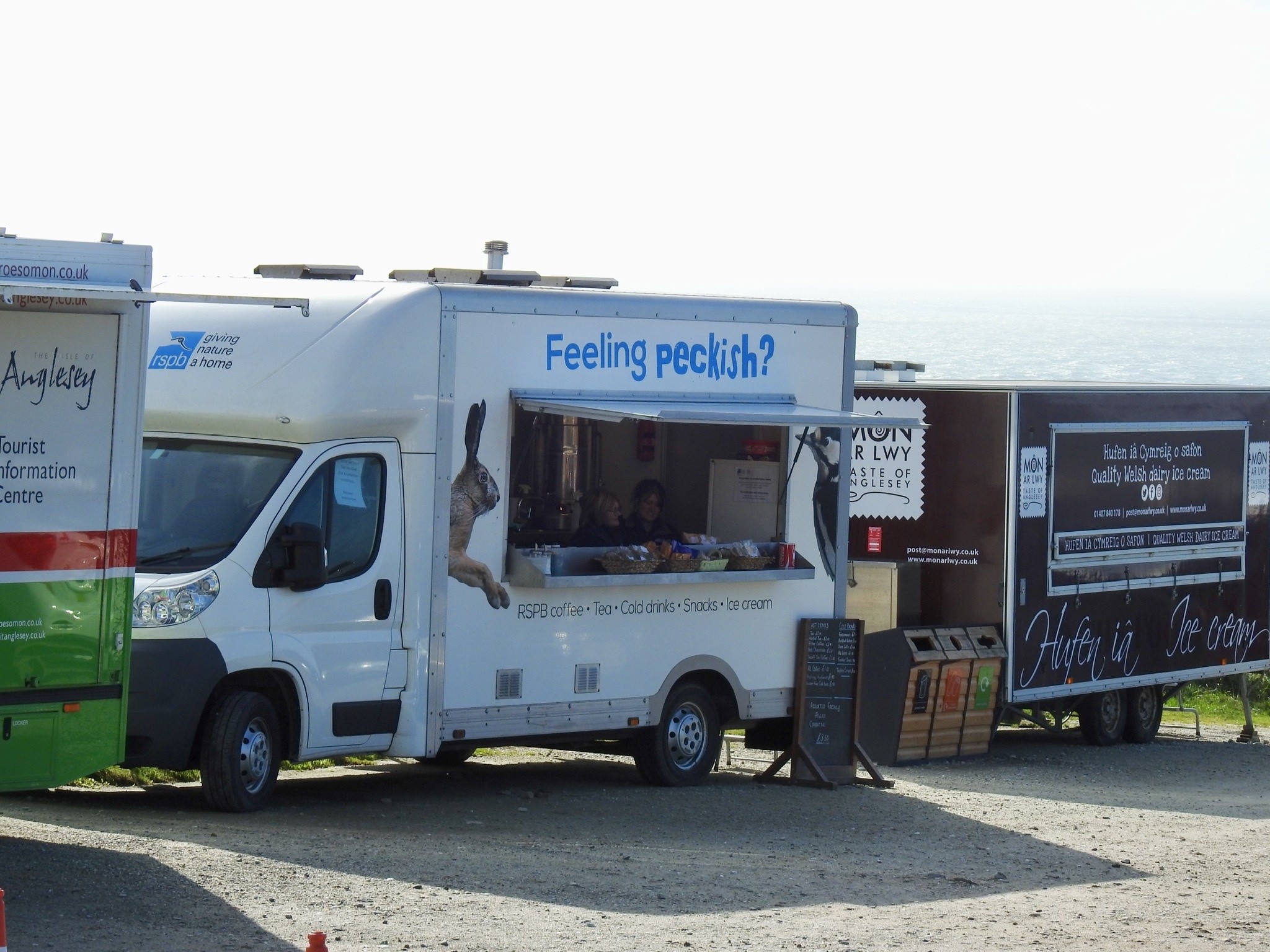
[859,621,1009,766]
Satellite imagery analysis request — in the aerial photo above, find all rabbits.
[449,398,511,609]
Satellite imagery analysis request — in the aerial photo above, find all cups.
[779,542,795,569]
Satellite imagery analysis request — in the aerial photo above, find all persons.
[568,491,632,546]
[622,476,680,547]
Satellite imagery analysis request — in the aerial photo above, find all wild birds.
[795,427,837,581]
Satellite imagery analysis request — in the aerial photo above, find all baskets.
[725,557,776,571]
[594,556,666,575]
[654,557,704,574]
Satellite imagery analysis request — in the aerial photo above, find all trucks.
[0,227,155,791]
[843,381,1270,746]
[123,280,862,805]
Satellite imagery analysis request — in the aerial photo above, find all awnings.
[511,389,932,428]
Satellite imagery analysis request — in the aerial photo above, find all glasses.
[607,506,620,513]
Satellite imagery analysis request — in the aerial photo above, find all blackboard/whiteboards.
[791,617,866,786]
